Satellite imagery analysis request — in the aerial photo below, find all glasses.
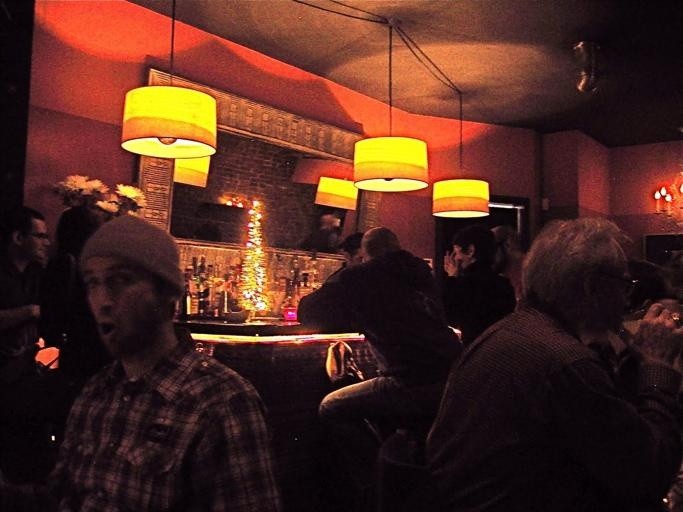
[591,266,642,298]
[17,229,51,241]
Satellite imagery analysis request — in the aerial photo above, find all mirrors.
[168,129,359,261]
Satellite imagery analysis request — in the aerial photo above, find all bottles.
[175,255,241,318]
[268,254,323,316]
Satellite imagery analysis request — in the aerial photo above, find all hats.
[79,211,188,302]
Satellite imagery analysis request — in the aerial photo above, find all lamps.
[351,26,431,194]
[432,89,490,219]
[119,1,217,160]
[311,176,358,212]
[172,154,211,187]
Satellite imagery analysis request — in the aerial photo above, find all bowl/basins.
[225,311,246,323]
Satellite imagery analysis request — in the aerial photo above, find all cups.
[621,305,646,334]
